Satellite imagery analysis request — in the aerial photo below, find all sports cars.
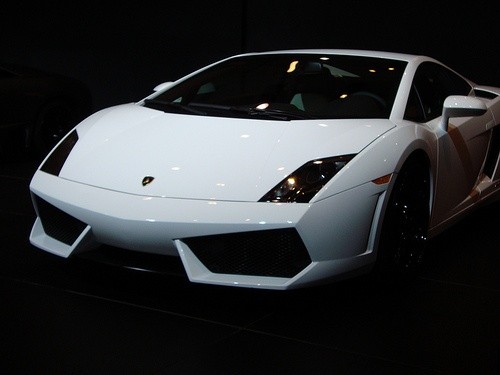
[26,47,499,290]
